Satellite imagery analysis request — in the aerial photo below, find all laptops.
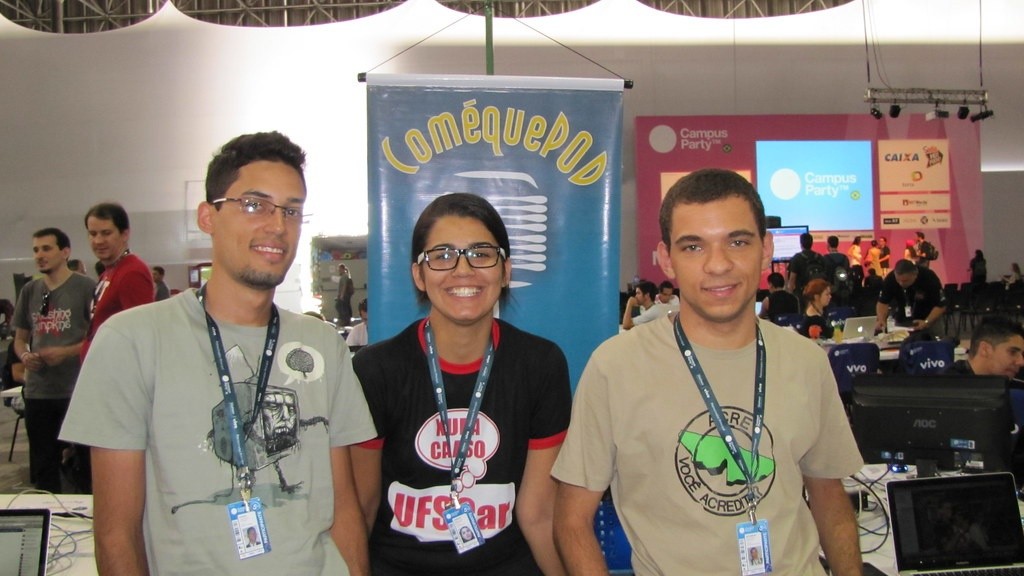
[886,472,1024,576]
[843,317,877,340]
[0,508,51,576]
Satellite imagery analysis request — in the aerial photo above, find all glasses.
[416,246,506,271]
[212,195,313,224]
[40,291,49,316]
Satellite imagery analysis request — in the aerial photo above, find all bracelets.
[21,351,29,359]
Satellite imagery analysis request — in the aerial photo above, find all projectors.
[926,111,948,121]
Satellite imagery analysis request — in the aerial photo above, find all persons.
[0,202,171,494]
[460,526,475,543]
[349,193,572,576]
[304,264,370,351]
[760,232,1024,377]
[246,527,260,549]
[550,169,870,576]
[57,130,379,576]
[748,547,762,565]
[622,280,680,333]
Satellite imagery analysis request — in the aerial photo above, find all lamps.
[867,98,995,123]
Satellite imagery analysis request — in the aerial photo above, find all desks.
[809,464,1024,576]
[0,492,99,576]
[818,341,969,366]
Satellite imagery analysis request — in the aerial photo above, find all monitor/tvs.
[853,374,1008,477]
[766,225,808,262]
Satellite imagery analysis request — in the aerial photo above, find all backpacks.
[928,243,939,261]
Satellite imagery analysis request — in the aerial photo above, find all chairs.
[773,278,1024,395]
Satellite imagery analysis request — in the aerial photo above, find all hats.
[906,239,915,248]
